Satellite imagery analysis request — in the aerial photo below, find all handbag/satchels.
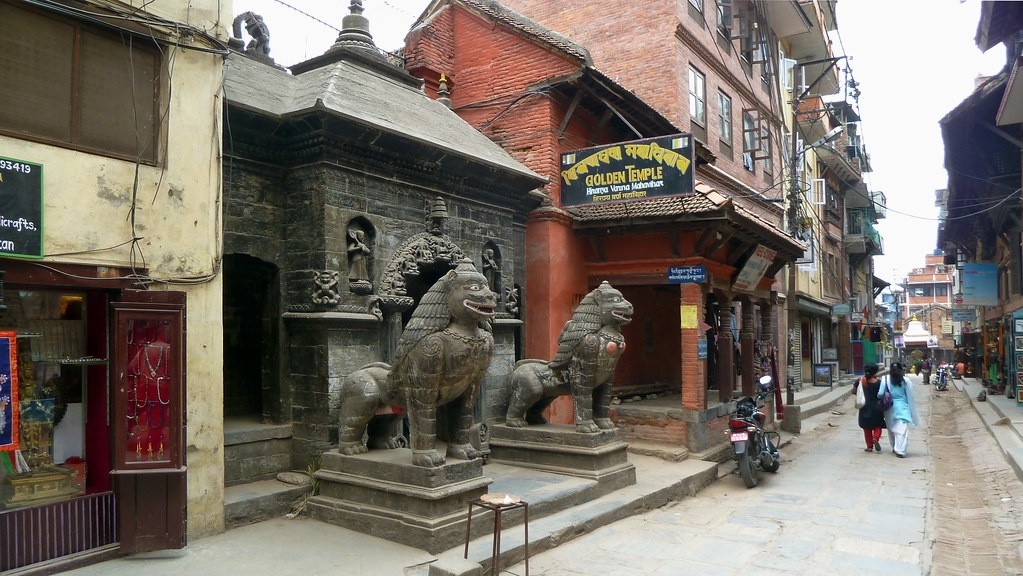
[878,386,893,411]
[854,378,866,410]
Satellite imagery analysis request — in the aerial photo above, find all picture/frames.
[814,364,832,387]
[821,346,838,381]
[1014,318,1023,403]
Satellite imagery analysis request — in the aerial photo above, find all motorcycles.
[722,375,782,487]
[932,365,948,391]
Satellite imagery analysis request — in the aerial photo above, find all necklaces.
[126,320,172,438]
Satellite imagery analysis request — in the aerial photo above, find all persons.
[919,354,965,385]
[852,362,887,453]
[877,363,920,458]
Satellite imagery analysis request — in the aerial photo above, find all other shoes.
[896,452,906,458]
[873,438,881,452]
[864,448,873,452]
